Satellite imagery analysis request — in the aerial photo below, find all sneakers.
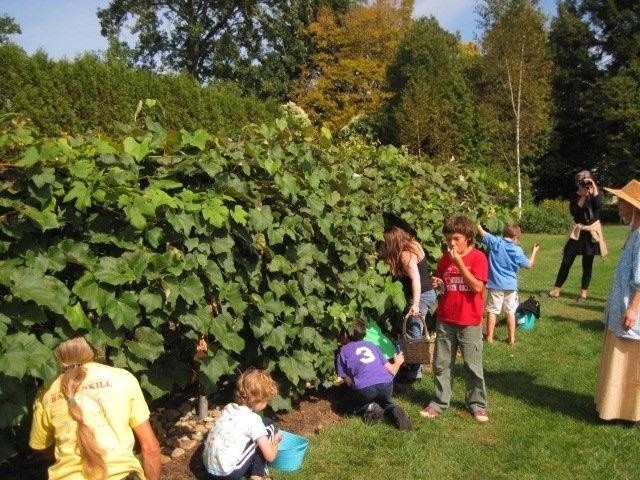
[385,404,412,429]
[248,474,273,480]
[364,402,384,423]
[473,409,489,422]
[419,406,439,418]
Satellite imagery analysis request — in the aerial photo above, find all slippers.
[539,291,560,298]
[576,296,586,303]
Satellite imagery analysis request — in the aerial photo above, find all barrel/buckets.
[514,310,535,330]
[267,429,309,473]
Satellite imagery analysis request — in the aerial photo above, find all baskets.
[399,312,435,364]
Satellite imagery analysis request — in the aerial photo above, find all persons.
[337,318,414,433]
[26,333,164,480]
[476,220,541,349]
[198,366,284,480]
[377,221,439,383]
[418,215,490,424]
[592,178,640,427]
[546,169,609,303]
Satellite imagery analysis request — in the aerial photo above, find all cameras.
[579,179,593,187]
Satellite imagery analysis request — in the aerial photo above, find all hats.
[603,179,640,211]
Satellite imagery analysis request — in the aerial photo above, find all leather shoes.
[394,374,421,384]
